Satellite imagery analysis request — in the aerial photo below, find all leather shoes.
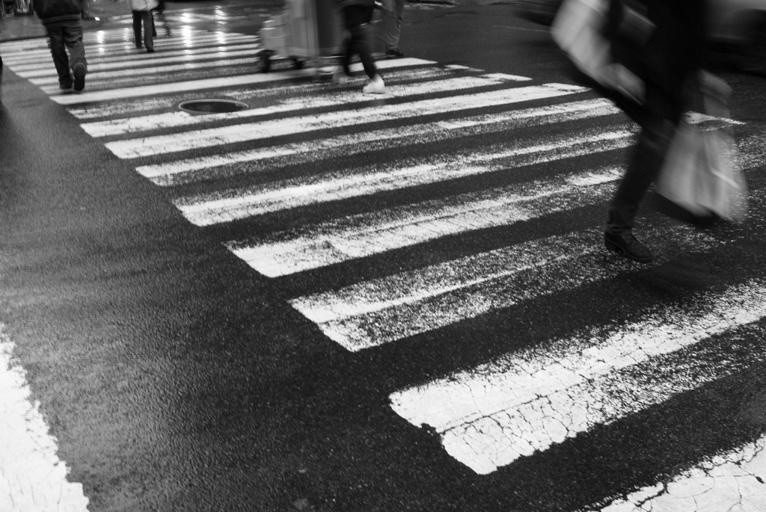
[604,232,652,263]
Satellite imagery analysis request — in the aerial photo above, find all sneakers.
[72,63,86,91]
[362,74,386,94]
[59,78,72,90]
[386,49,404,58]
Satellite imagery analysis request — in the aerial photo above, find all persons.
[32,0,90,90]
[549,0,707,261]
[364,0,406,58]
[131,0,158,52]
[151,0,171,37]
[333,0,385,94]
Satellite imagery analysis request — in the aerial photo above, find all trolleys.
[256,0,387,72]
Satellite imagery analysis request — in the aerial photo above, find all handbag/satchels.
[653,72,747,231]
